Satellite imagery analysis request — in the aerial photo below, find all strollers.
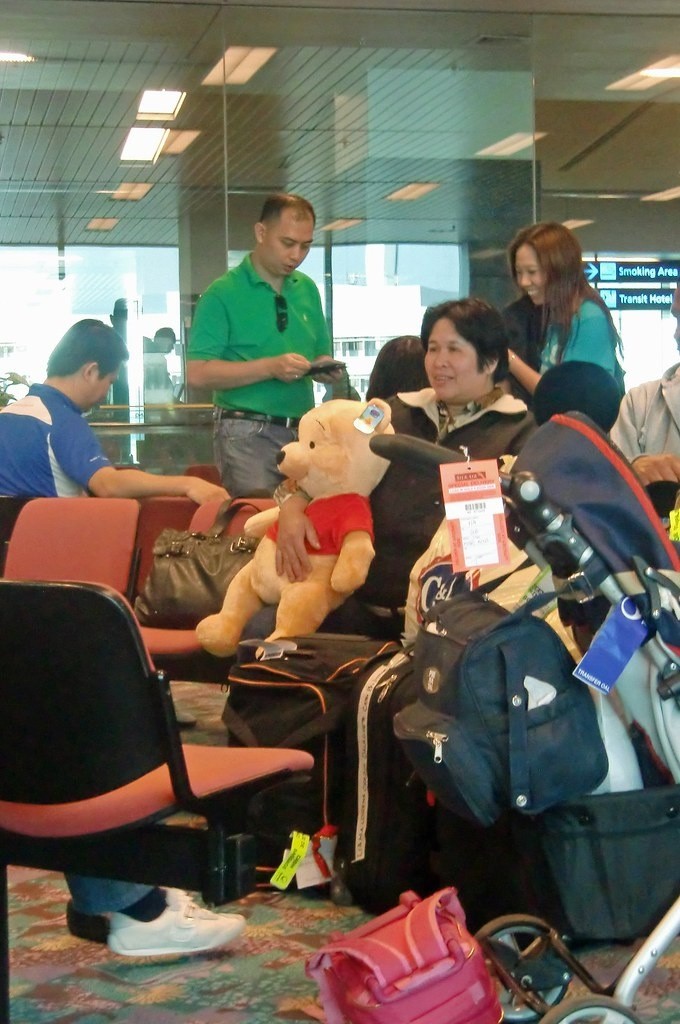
[369,410,680,1024]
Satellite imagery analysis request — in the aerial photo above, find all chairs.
[0,581,313,906]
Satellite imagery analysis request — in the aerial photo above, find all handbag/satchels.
[133,529,280,639]
[221,637,401,883]
[524,786,680,947]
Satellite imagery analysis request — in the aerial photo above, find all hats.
[527,362,620,431]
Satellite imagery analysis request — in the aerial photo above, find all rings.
[295,375,298,379]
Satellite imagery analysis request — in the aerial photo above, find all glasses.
[274,293,289,333]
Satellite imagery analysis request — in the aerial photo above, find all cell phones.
[305,364,346,375]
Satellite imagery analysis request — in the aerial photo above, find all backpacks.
[392,593,610,824]
[311,899,504,1024]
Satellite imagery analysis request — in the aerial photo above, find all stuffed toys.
[196,398,395,659]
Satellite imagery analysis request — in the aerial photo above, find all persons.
[609,284,680,487]
[109,300,176,423]
[273,298,540,640]
[508,220,626,399]
[0,320,233,506]
[64,872,246,956]
[186,193,343,499]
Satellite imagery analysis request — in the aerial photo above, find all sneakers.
[107,888,245,953]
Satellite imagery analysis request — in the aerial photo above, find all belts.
[212,407,302,427]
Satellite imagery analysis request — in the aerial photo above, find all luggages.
[343,647,428,913]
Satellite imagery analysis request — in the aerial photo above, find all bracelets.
[509,354,516,364]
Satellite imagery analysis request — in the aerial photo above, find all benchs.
[0,496,285,684]
